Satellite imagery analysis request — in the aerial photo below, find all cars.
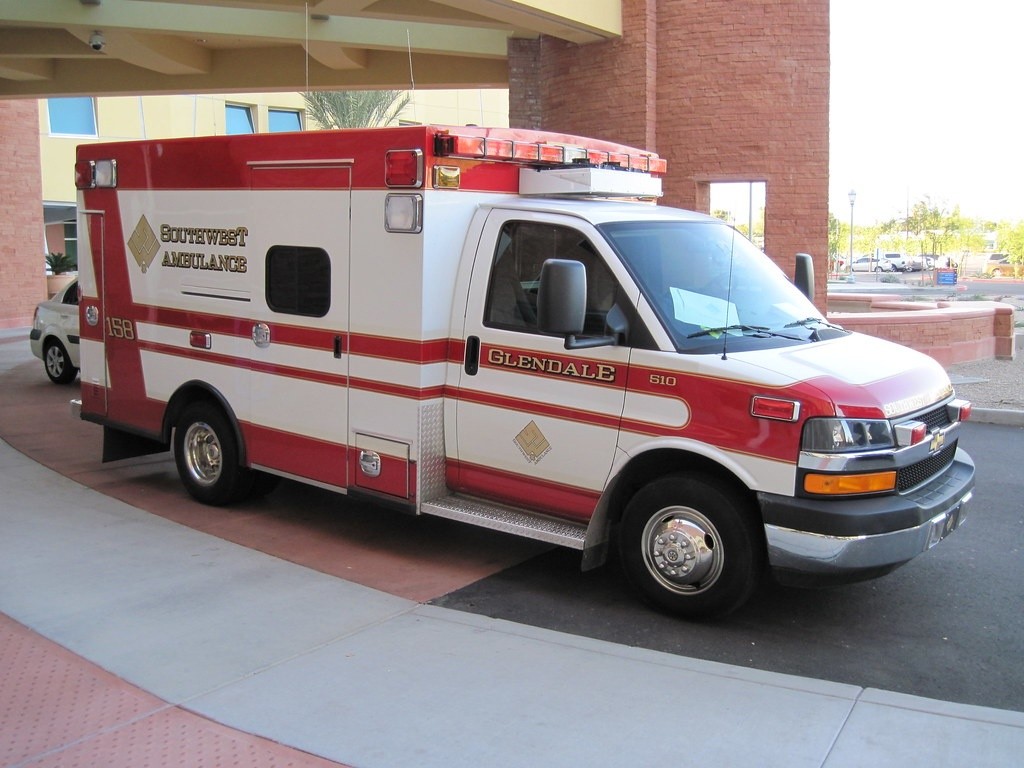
[27,274,80,386]
[846,250,1016,278]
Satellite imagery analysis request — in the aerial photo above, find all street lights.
[845,188,857,284]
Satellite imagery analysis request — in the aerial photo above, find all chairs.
[488,246,538,329]
[559,230,618,316]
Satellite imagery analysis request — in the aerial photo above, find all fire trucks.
[73,123,978,627]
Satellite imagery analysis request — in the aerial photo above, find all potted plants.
[46,252,78,294]
